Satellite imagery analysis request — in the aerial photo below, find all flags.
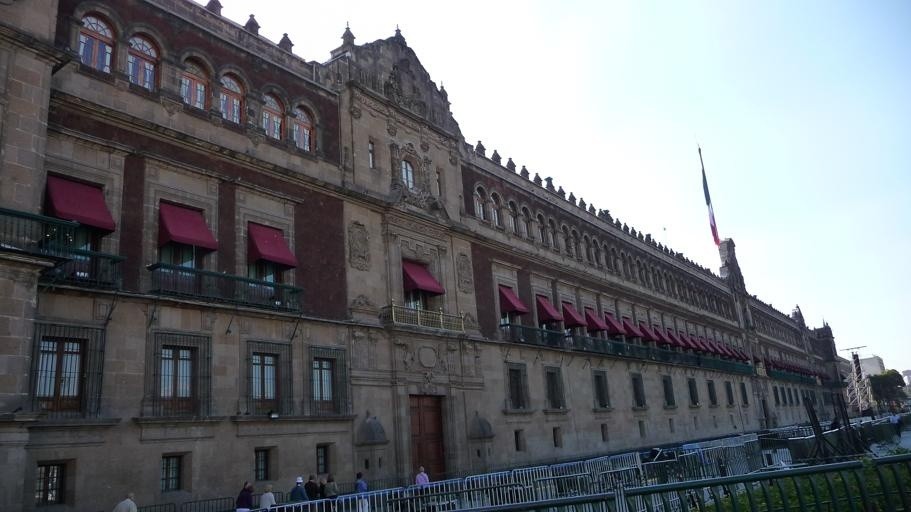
[702,168,720,246]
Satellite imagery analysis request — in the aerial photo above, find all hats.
[296,477,304,483]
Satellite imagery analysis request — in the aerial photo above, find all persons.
[235,473,339,512]
[354,472,368,512]
[890,411,901,437]
[112,492,137,512]
[415,466,429,484]
